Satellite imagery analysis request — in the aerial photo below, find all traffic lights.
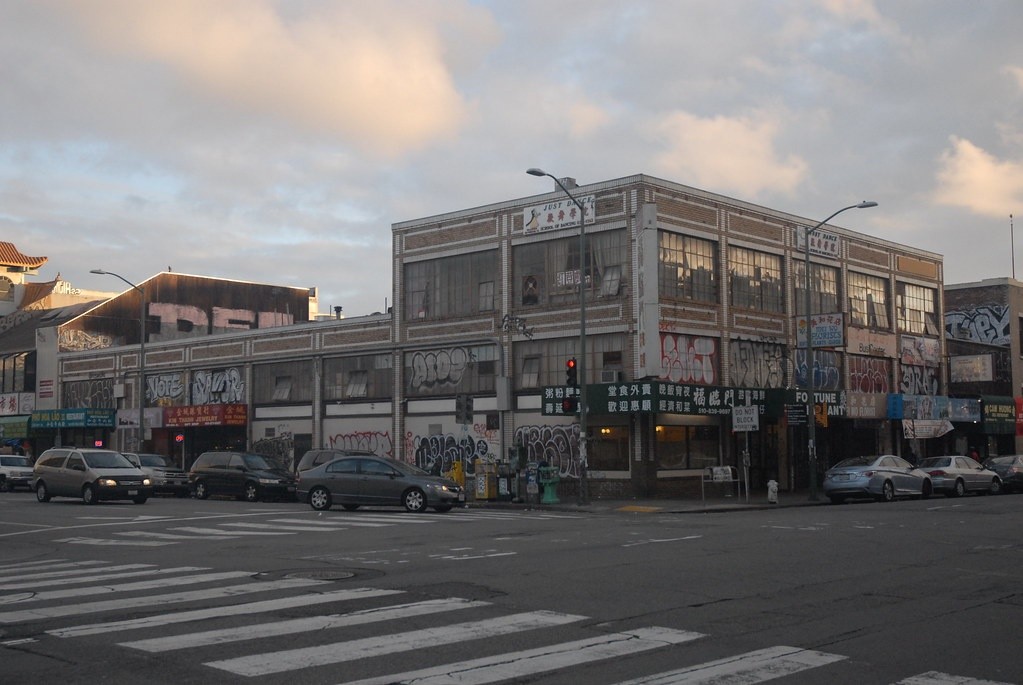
[563,398,580,416]
[566,358,578,388]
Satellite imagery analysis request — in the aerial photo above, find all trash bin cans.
[494,459,513,501]
[540,467,559,503]
[474,458,496,501]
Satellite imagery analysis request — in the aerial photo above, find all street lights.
[523,167,591,510]
[90,268,147,454]
[803,201,881,504]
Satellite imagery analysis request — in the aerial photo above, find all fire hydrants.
[767,481,778,506]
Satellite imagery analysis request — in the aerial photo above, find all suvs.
[120,453,189,499]
[189,450,300,503]
[31,445,153,506]
[295,447,386,502]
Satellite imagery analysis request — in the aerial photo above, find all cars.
[982,455,1023,495]
[296,456,467,514]
[0,455,38,494]
[915,456,1004,499]
[822,455,933,503]
